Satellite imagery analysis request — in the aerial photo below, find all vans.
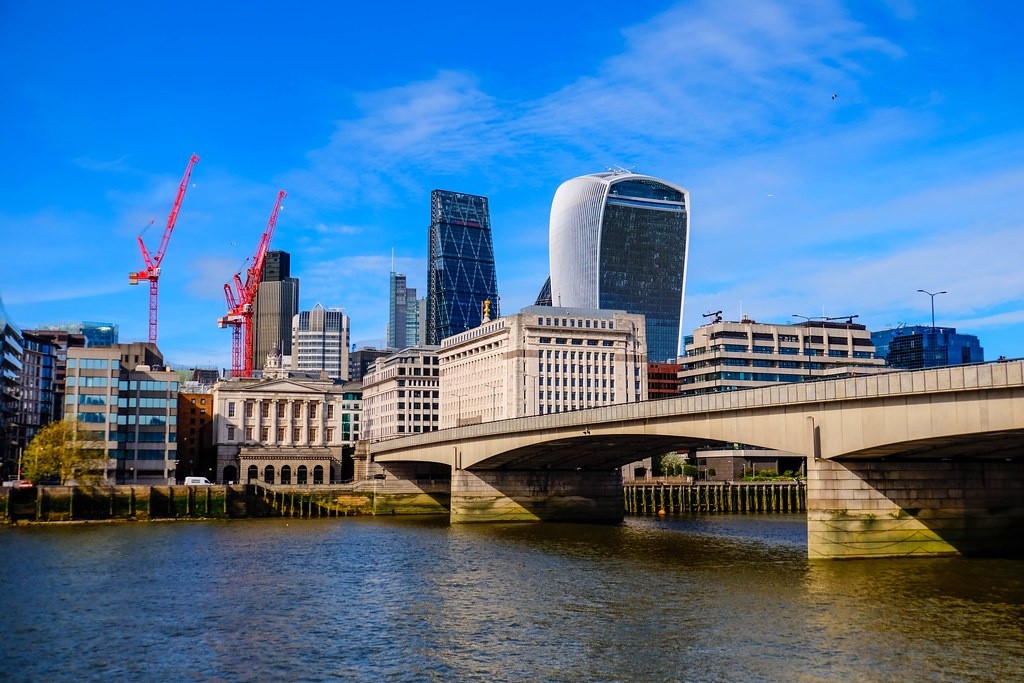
[184,477,215,486]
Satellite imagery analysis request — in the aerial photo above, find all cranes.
[216,190,287,379]
[129,156,199,345]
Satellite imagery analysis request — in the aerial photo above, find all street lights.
[523,374,544,416]
[377,415,388,442]
[917,290,948,367]
[451,393,468,427]
[705,469,708,481]
[743,464,745,477]
[630,350,654,401]
[485,384,502,421]
[792,314,828,381]
[702,334,727,391]
[753,463,756,477]
